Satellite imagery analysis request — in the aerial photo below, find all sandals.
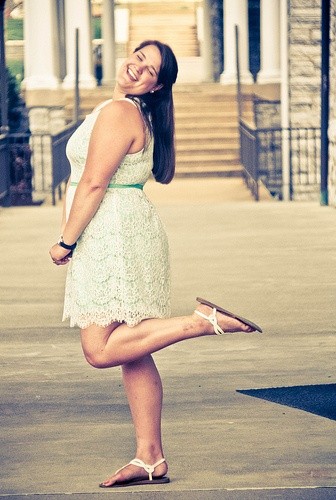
[194,296,263,336]
[99,457,170,488]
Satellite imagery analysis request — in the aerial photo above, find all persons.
[49,39,262,487]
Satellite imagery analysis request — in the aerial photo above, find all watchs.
[58,235,77,250]
